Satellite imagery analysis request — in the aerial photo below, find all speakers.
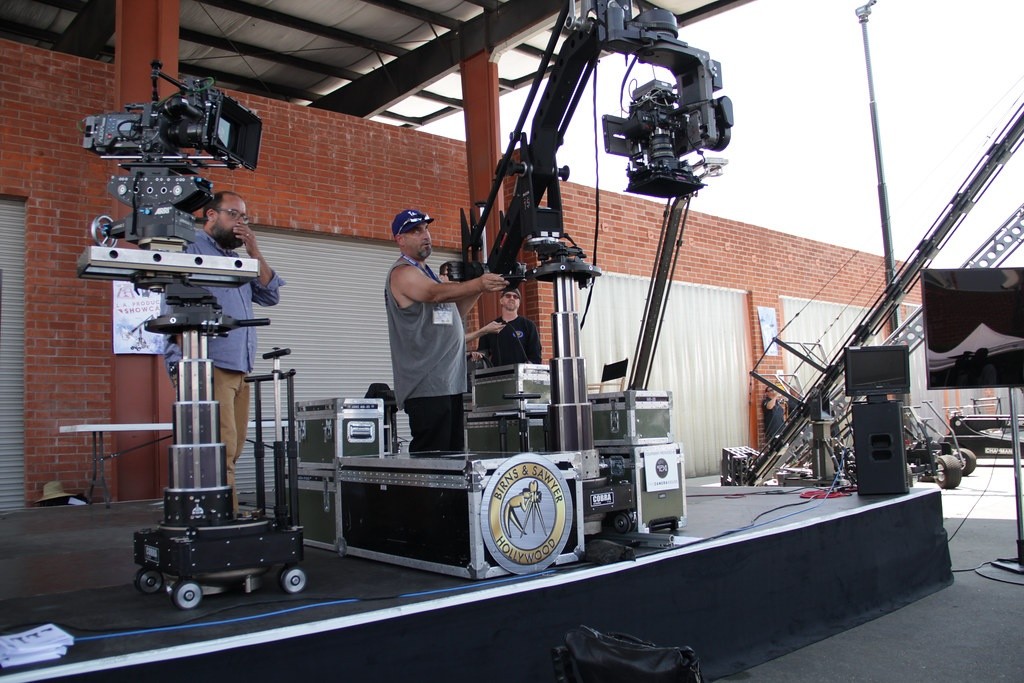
[852,400,910,495]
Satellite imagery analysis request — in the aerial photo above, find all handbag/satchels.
[553,625,704,683]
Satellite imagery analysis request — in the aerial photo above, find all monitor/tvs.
[920,266,1024,391]
[844,344,910,403]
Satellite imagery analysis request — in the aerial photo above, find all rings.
[246,233,249,237]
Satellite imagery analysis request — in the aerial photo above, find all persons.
[787,386,802,416]
[159,190,286,522]
[761,384,787,448]
[461,287,544,369]
[384,209,510,460]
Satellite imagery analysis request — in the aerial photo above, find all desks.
[58,420,291,509]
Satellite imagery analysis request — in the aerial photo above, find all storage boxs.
[286,364,687,581]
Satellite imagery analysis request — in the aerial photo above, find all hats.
[392,210,434,234]
[501,288,521,299]
[34,481,77,503]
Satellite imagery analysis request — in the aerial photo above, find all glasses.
[398,214,430,234]
[503,294,520,299]
[215,209,250,224]
[767,389,773,392]
